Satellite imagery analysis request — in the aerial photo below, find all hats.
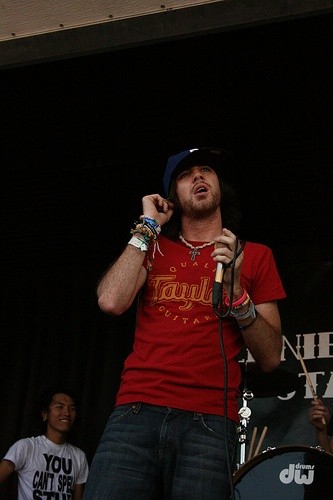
[163,147,224,197]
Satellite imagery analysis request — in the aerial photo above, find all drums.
[232,444,333,500]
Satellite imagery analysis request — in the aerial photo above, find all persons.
[308,399,333,454]
[0,389,89,500]
[80,148,287,500]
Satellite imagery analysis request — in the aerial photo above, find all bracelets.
[225,288,258,330]
[127,214,164,258]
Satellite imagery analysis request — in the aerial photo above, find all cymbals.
[236,363,301,399]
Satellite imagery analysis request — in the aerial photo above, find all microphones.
[212,241,231,309]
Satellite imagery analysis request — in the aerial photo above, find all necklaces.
[178,229,215,260]
